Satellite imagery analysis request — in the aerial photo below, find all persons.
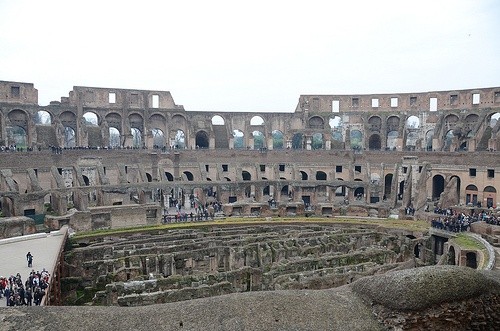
[0,143,292,155]
[160,196,223,225]
[251,189,430,219]
[0,251,51,308]
[430,193,500,235]
[352,143,499,153]
